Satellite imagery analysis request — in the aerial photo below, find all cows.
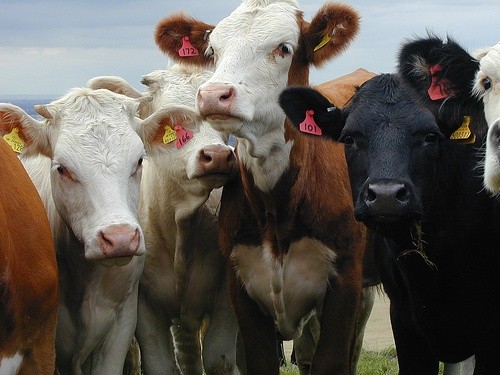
[1,86,198,374]
[85,62,245,374]
[276,27,499,374]
[152,1,378,374]
